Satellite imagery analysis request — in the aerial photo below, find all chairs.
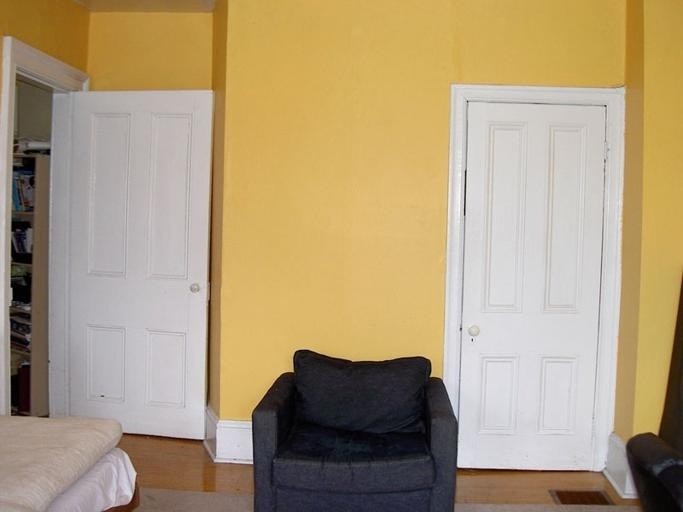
[251,373,458,512]
[626,433,683,512]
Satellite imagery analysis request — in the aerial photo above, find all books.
[8,156,35,417]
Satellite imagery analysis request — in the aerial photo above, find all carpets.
[130,480,638,512]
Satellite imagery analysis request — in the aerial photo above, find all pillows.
[294,351,432,433]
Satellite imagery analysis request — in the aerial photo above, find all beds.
[0,415,138,512]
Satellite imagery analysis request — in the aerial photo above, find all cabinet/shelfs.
[10,152,50,417]
